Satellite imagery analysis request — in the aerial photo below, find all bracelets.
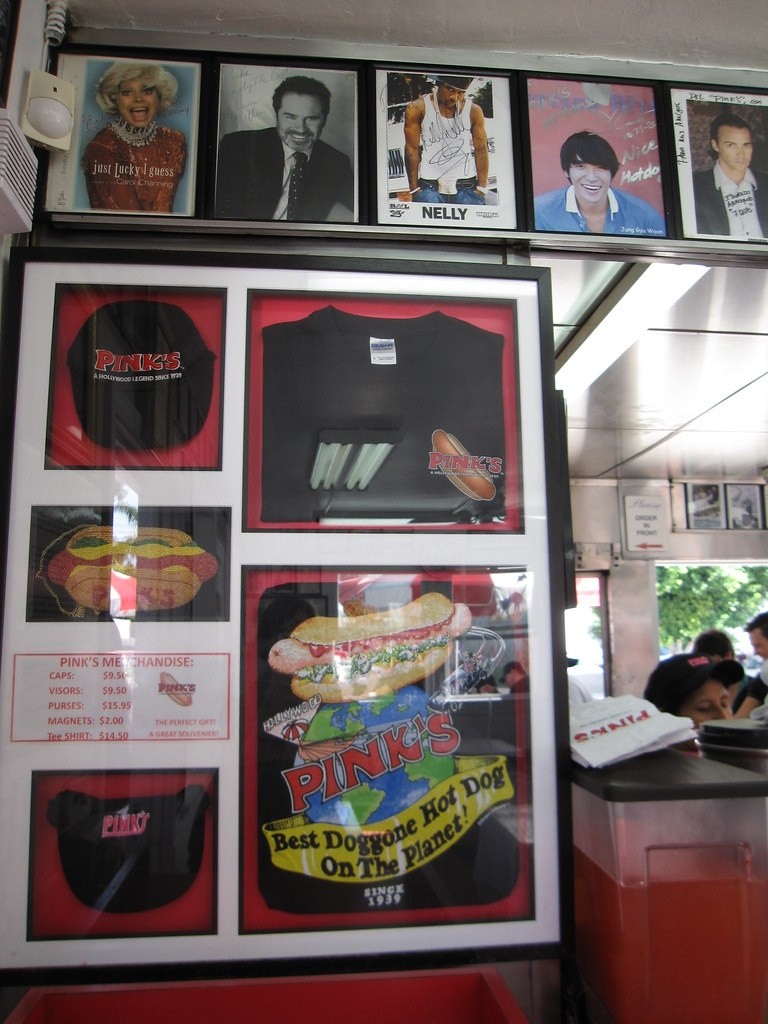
[409,186,421,194]
[476,185,489,195]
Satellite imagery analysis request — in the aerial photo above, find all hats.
[643,654,744,719]
[429,75,472,91]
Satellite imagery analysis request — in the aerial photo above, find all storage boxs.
[3,965,529,1024]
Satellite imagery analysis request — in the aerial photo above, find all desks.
[570,748,768,1024]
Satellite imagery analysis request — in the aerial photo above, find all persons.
[461,652,498,693]
[565,654,594,704]
[218,74,354,221]
[534,129,666,238]
[693,630,755,715]
[643,653,745,729]
[693,111,768,239]
[499,659,529,693]
[81,57,188,211]
[734,612,768,720]
[399,76,491,205]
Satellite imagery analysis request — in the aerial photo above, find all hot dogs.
[268,592,475,703]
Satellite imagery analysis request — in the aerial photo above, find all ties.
[286,152,308,222]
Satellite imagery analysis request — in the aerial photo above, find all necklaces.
[108,116,159,148]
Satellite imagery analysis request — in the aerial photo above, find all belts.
[416,178,478,190]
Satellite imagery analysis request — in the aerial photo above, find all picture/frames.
[0,41,768,986]
